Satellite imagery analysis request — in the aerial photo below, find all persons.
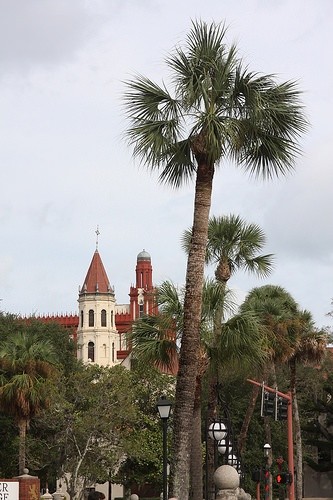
[86,490,107,500]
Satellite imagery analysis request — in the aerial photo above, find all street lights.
[209,414,232,467]
[157,394,172,500]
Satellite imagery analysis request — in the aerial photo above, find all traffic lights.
[260,388,274,418]
[275,392,287,420]
